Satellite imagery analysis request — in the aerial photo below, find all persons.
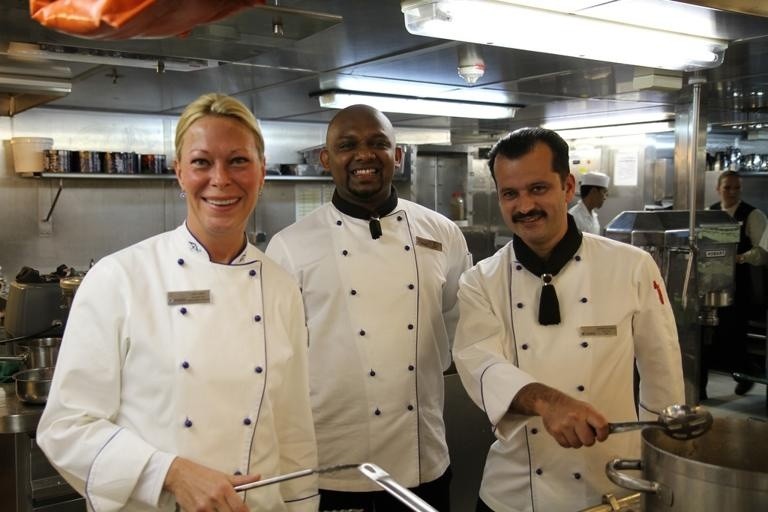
[34,92,323,512]
[703,170,768,396]
[264,104,474,512]
[450,126,686,512]
[566,170,611,236]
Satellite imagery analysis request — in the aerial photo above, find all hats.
[580,171,611,188]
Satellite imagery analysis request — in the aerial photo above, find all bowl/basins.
[277,162,324,176]
[9,135,54,177]
[13,368,58,402]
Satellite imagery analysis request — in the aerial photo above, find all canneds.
[44,150,166,173]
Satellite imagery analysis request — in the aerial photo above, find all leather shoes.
[735,381,753,395]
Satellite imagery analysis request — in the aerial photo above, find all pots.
[605,410,767,511]
[0,338,60,369]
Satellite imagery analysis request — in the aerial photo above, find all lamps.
[395,0,729,71]
[312,88,526,127]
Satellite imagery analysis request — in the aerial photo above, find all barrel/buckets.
[10,137,52,172]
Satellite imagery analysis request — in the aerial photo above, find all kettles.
[705,146,768,172]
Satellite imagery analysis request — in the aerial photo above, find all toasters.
[4,266,59,337]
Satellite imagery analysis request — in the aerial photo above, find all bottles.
[451,192,466,219]
